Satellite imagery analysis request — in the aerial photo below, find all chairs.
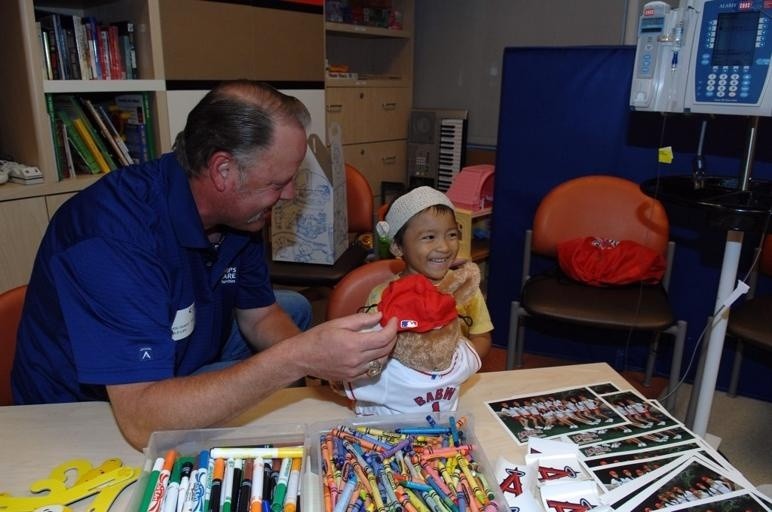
[344,162,376,234]
[326,259,407,321]
[0,284,30,406]
[505,175,687,414]
[721,234,772,398]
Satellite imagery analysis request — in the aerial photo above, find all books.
[34,12,155,184]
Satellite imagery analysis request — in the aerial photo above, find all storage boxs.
[271,120,350,265]
[160,0,324,82]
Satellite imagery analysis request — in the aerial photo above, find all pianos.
[438,118,466,193]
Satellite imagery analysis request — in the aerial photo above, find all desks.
[266,246,368,289]
[0,361,772,512]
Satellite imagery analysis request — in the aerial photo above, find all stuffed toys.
[327,273,482,426]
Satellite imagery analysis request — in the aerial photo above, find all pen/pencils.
[319,416,501,512]
[139,442,305,512]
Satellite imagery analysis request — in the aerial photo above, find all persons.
[364,183,495,360]
[498,391,734,512]
[10,80,399,455]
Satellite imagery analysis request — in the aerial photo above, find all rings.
[368,360,382,378]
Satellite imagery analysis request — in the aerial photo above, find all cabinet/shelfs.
[324,0,413,240]
[0,0,173,201]
[0,190,82,295]
